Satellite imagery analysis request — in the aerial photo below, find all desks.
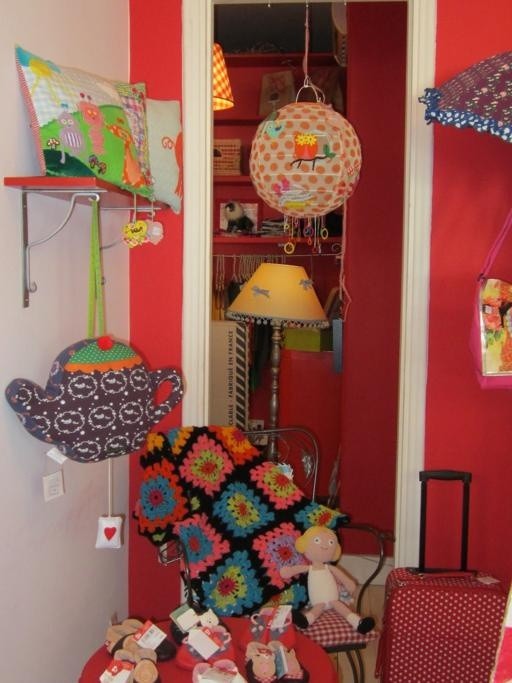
[78,617,341,683]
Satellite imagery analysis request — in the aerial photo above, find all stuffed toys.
[279,524,376,633]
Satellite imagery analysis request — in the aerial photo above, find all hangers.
[213,255,288,293]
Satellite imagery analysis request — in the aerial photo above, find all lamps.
[212,42,236,156]
[227,264,331,462]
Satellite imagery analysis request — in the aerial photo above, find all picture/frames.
[215,198,264,236]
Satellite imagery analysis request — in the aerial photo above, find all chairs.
[180,427,385,682]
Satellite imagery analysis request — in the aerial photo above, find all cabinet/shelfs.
[212,51,344,244]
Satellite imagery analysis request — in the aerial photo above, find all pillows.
[14,44,158,203]
[146,96,184,215]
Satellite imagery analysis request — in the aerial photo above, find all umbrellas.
[418,48,511,145]
